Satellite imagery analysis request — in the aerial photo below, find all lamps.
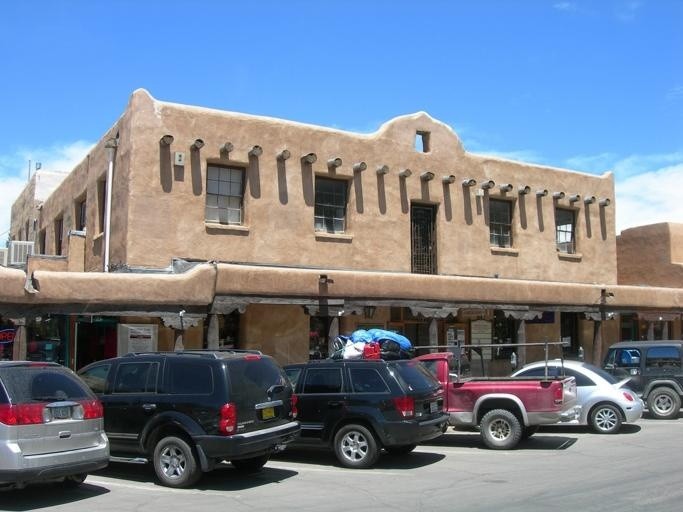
[364,305,377,320]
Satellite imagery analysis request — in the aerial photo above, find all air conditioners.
[5,238,37,267]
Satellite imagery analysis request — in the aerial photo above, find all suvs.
[0,360,110,493]
[272,356,451,470]
[76,349,303,489]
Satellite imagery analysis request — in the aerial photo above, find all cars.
[508,340,683,434]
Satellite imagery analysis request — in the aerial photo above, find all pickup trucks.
[411,352,582,450]
